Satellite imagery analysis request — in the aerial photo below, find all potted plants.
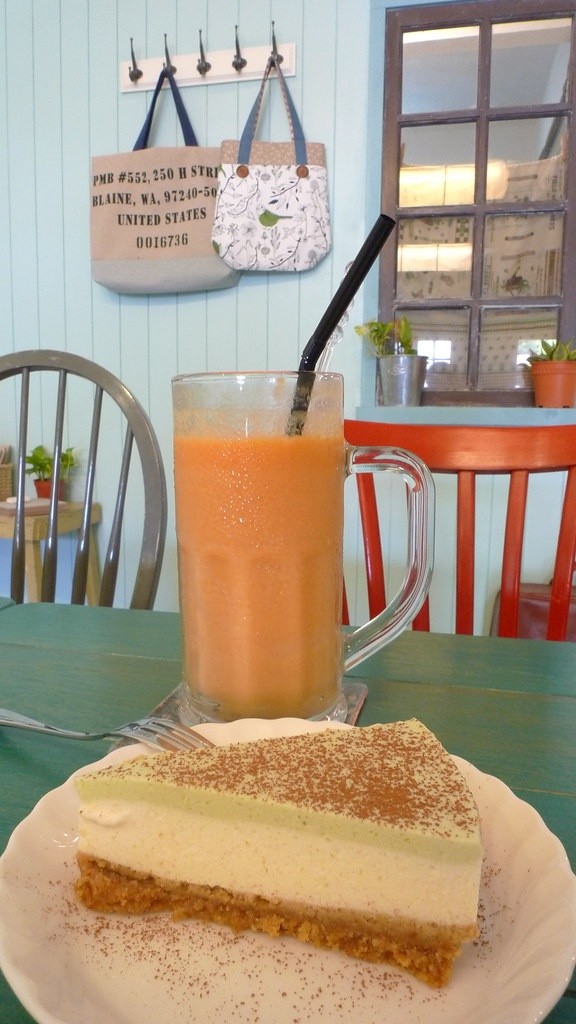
[355,317,429,409]
[22,444,78,503]
[524,339,576,410]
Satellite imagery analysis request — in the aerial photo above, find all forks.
[0,706,220,756]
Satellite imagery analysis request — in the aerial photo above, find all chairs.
[0,349,172,610]
[344,419,576,641]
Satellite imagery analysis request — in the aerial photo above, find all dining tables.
[0,597,575,1024]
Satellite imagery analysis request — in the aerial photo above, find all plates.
[0,713,574,1024]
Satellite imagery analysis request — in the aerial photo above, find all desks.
[0,500,102,606]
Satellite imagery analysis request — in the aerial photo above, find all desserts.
[77,715,486,990]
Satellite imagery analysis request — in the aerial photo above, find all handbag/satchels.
[89,56,331,300]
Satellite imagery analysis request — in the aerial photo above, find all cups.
[167,370,438,736]
